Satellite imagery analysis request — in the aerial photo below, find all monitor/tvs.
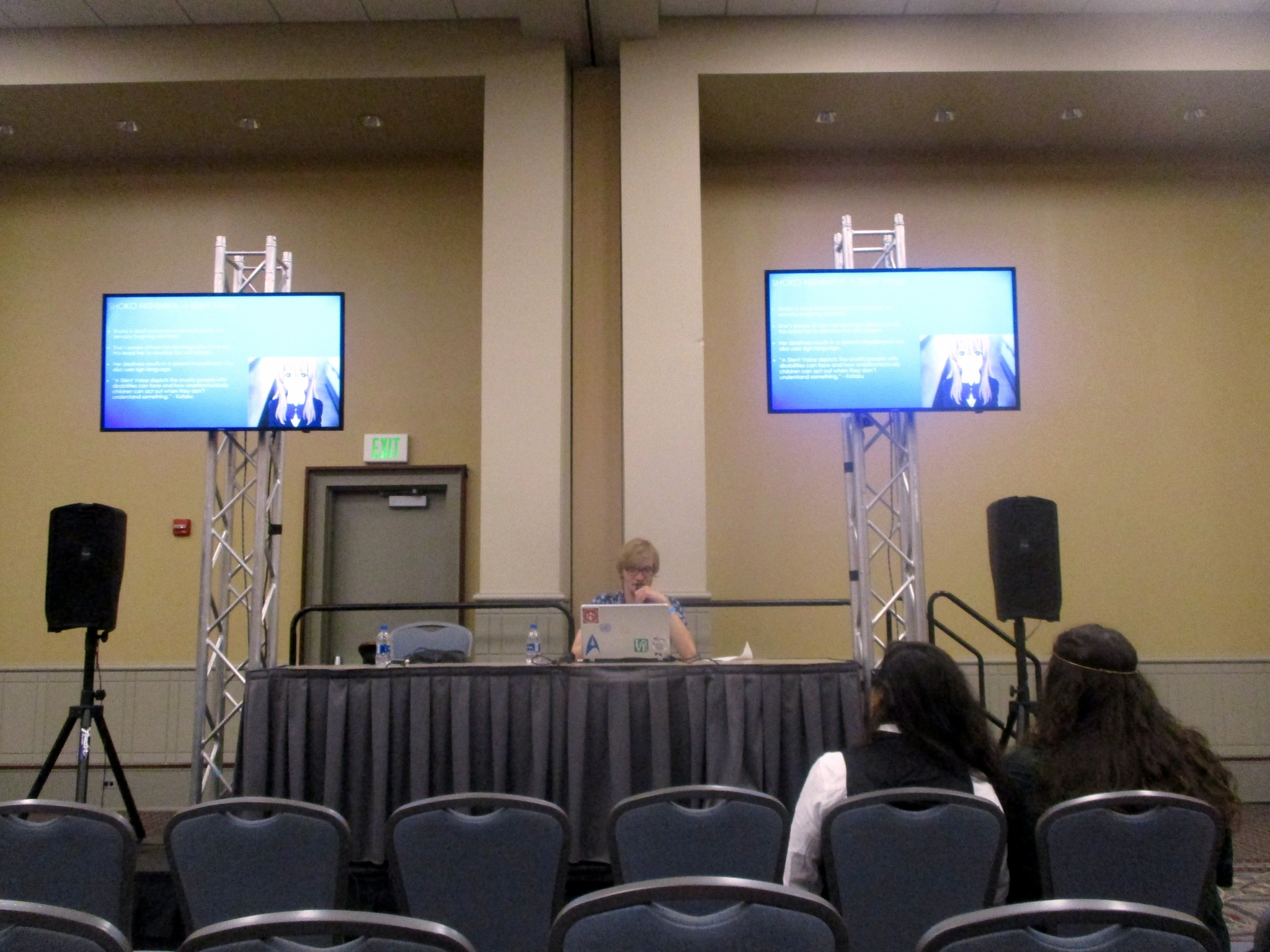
[765,267,1023,412]
[100,292,346,433]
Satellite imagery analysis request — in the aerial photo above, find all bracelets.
[668,607,676,615]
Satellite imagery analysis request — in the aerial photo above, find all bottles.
[526,624,540,665]
[375,625,391,666]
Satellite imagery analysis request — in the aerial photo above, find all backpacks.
[407,647,470,664]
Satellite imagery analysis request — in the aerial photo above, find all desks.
[235,657,863,867]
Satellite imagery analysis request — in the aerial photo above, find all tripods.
[13,628,146,840]
[996,617,1042,753]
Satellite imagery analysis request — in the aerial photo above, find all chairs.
[391,621,474,661]
[0,784,1229,952]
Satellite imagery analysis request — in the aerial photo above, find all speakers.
[986,496,1061,622]
[45,504,128,632]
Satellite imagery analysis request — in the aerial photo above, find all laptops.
[580,603,676,663]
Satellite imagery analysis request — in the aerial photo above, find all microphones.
[636,583,649,604]
[552,652,576,665]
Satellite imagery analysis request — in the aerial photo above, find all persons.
[571,537,697,660]
[1004,624,1239,952]
[781,640,1010,908]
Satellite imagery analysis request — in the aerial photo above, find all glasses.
[870,667,882,686]
[625,565,654,577]
